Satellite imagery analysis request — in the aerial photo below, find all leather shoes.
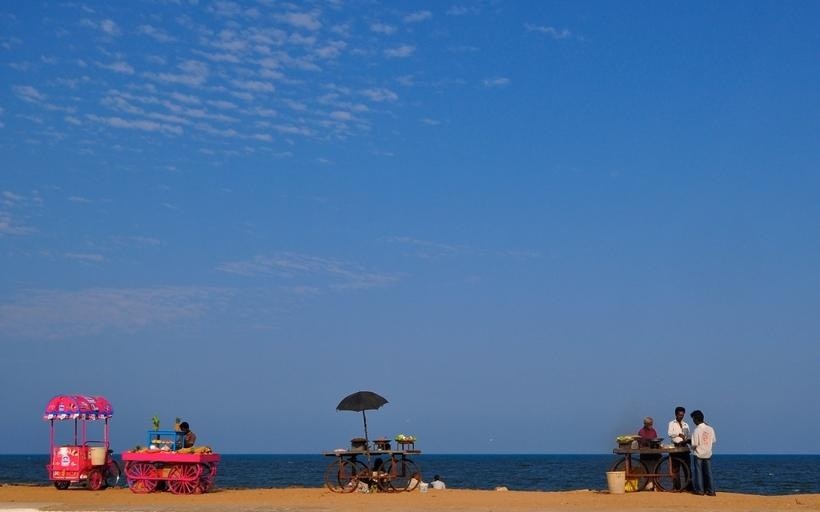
[692,490,704,495]
[705,490,715,496]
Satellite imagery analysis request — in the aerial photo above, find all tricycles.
[45,395,121,490]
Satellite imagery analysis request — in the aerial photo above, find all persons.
[668,406,694,492]
[408,472,424,488]
[430,475,445,488]
[638,416,660,491]
[689,410,717,496]
[366,457,387,491]
[170,422,196,451]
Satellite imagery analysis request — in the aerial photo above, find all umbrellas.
[336,391,390,451]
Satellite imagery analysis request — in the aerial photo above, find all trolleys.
[323,447,423,493]
[120,428,219,495]
[608,444,693,494]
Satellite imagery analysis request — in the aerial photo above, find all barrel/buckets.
[90,446,107,465]
[605,471,626,494]
[419,482,429,492]
